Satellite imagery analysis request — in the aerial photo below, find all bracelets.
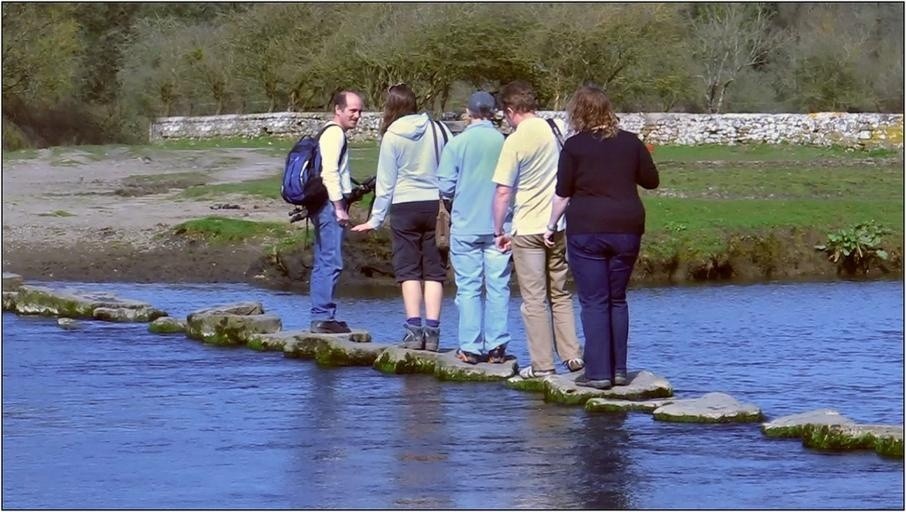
[547,224,558,232]
[493,229,506,237]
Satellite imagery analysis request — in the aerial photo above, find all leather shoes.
[310,320,350,334]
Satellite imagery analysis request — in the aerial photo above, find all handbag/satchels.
[436,198,451,249]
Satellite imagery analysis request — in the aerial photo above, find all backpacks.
[281,125,346,204]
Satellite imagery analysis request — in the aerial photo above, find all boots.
[399,321,439,350]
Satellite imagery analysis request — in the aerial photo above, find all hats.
[468,92,494,115]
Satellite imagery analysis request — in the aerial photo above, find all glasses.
[388,82,409,94]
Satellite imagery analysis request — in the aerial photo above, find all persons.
[435,89,519,363]
[543,84,659,389]
[490,77,585,379]
[305,91,363,333]
[350,83,455,351]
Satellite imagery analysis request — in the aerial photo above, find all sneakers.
[519,365,557,379]
[567,358,585,371]
[573,372,626,388]
[486,348,506,362]
[457,348,479,364]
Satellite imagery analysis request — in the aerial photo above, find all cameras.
[343,176,376,207]
